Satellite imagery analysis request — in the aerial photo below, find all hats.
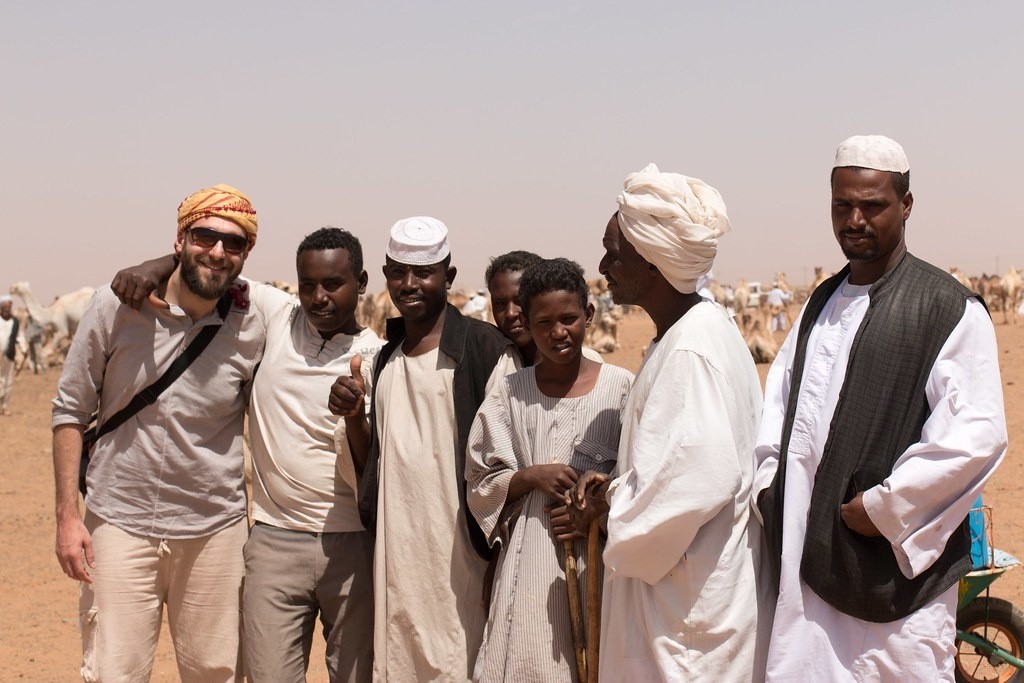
[0,295,13,305]
[617,162,731,294]
[834,134,910,174]
[387,216,450,265]
[178,183,258,259]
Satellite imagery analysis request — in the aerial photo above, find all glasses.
[184,226,248,254]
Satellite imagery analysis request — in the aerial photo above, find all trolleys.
[954,505,1024,682]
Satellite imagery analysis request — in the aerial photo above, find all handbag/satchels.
[79,435,93,494]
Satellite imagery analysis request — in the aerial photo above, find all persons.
[596,280,621,351]
[485,251,603,369]
[328,217,506,683]
[0,297,47,415]
[52,184,266,683]
[465,290,488,322]
[566,165,777,683]
[769,284,792,333]
[750,136,1009,683]
[111,227,387,683]
[464,258,634,683]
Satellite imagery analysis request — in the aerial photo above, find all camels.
[711,266,825,365]
[264,281,470,339]
[948,266,1024,324]
[9,281,96,378]
[585,277,642,354]
